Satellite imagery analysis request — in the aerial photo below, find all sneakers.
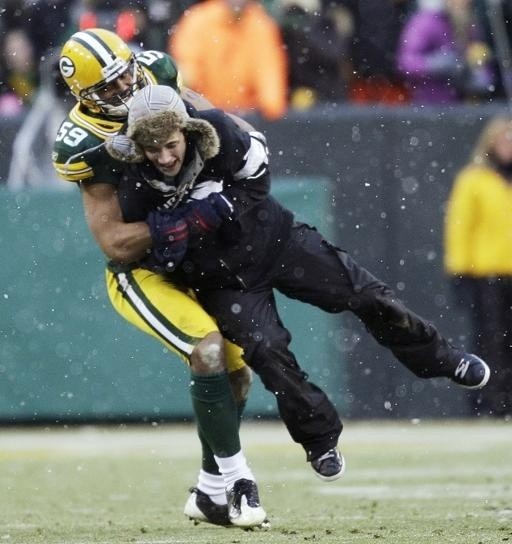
[226,477,267,526]
[185,486,270,531]
[309,446,345,481]
[449,352,490,390]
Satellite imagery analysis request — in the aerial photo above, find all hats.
[106,84,220,163]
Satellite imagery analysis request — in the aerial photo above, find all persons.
[49,28,268,529]
[116,84,490,481]
[440,110,512,424]
[1,0,512,116]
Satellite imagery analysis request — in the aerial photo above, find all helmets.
[59,27,149,121]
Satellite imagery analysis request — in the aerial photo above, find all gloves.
[185,191,239,248]
[147,210,190,272]
